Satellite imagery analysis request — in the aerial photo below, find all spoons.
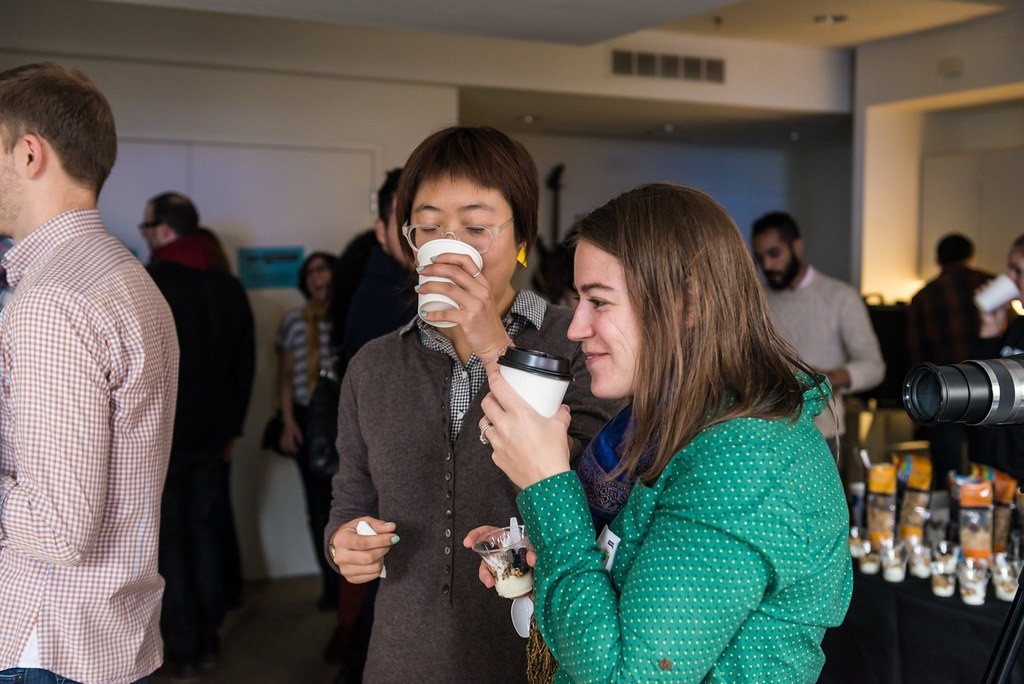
[510,517,534,637]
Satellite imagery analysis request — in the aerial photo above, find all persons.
[462,184,854,683]
[973,231,1024,483]
[324,169,416,666]
[275,252,338,609]
[141,193,257,683]
[0,60,180,684]
[325,128,628,684]
[904,234,999,465]
[750,212,886,479]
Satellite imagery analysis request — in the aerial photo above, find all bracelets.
[328,544,336,557]
[482,339,512,365]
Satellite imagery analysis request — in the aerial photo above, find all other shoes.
[154,665,200,684]
[324,624,353,664]
[201,654,218,673]
[317,583,339,611]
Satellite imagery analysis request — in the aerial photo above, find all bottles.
[957,481,1016,570]
[865,461,898,553]
[897,458,931,543]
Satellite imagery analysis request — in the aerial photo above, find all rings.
[473,271,481,278]
[479,424,492,444]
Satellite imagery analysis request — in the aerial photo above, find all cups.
[417,239,483,327]
[859,537,880,574]
[906,534,933,579]
[957,556,989,606]
[496,346,574,418]
[931,541,962,597]
[990,552,1024,602]
[879,538,909,582]
[471,525,534,599]
[848,482,866,526]
[973,273,1018,313]
[850,526,866,558]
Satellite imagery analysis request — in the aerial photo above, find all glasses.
[139,222,155,236]
[402,216,514,254]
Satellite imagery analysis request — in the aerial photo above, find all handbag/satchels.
[261,403,317,465]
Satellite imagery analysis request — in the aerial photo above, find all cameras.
[902,352,1024,426]
